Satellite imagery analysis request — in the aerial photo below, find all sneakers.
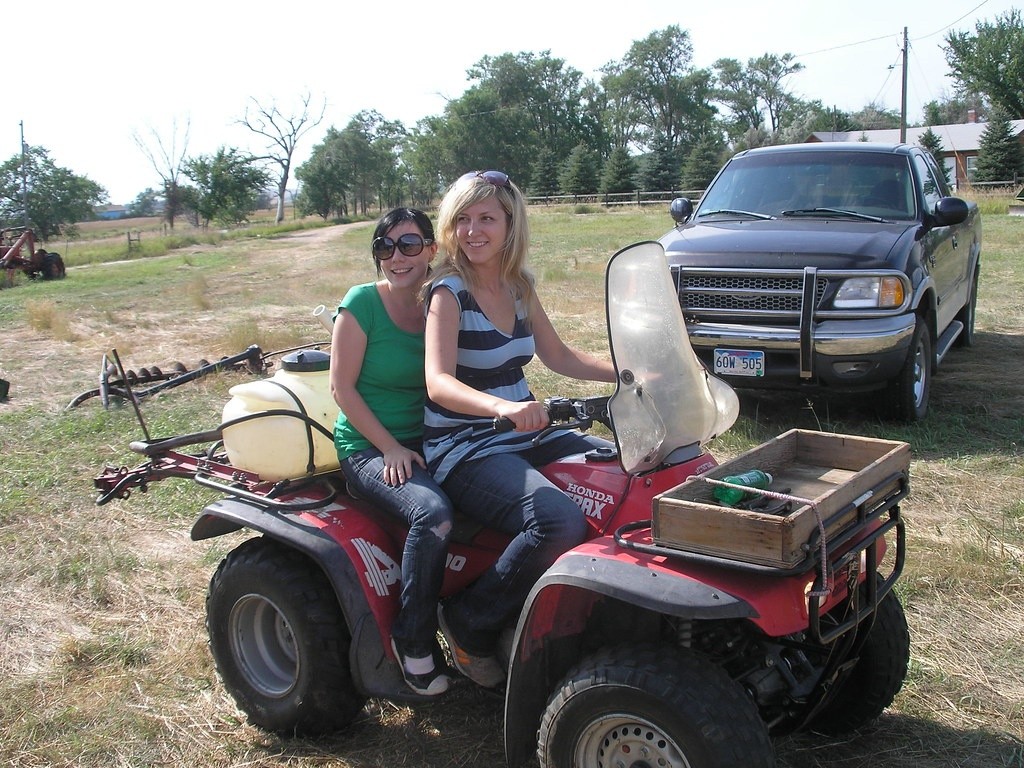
[437,603,506,689]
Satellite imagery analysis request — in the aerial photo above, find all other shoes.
[391,636,450,695]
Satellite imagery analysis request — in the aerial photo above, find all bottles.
[715,469,773,503]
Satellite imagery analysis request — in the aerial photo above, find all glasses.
[372,233,434,261]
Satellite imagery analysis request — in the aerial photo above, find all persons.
[415,169,665,689]
[330,206,448,696]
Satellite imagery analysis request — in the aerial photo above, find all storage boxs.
[652,427,911,567]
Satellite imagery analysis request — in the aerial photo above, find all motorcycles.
[92,237,911,767]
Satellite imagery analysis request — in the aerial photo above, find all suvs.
[652,141,983,425]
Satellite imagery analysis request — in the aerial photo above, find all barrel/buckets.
[220,350,338,481]
